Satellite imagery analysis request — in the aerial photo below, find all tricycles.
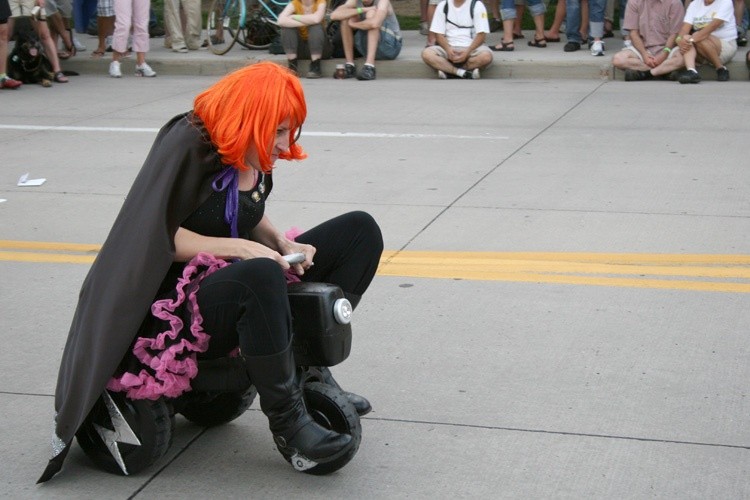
[74,251,363,476]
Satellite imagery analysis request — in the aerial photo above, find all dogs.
[5,30,81,85]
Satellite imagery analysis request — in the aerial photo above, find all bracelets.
[663,47,672,53]
[295,14,301,22]
[688,41,696,46]
[356,7,363,15]
[675,35,683,42]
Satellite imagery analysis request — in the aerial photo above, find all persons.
[36,59,384,487]
[276,0,332,78]
[420,0,750,84]
[0,0,156,89]
[162,0,232,54]
[330,0,403,81]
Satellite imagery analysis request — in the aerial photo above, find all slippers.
[544,34,560,42]
[64,29,77,57]
[513,33,524,39]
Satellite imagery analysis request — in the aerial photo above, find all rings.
[311,262,314,265]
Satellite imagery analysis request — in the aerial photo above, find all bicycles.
[205,0,341,55]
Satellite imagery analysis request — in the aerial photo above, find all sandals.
[528,37,545,48]
[488,40,513,51]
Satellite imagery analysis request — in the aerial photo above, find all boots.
[303,360,371,416]
[237,333,357,463]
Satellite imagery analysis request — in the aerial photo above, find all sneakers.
[333,64,356,79]
[135,63,157,78]
[289,60,298,72]
[359,64,375,80]
[0,76,22,88]
[108,60,122,77]
[307,61,321,78]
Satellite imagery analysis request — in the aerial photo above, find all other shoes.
[55,71,68,82]
[563,29,746,84]
[72,37,87,51]
[438,69,447,79]
[180,47,187,53]
[92,51,105,58]
[465,68,480,79]
[201,35,226,48]
